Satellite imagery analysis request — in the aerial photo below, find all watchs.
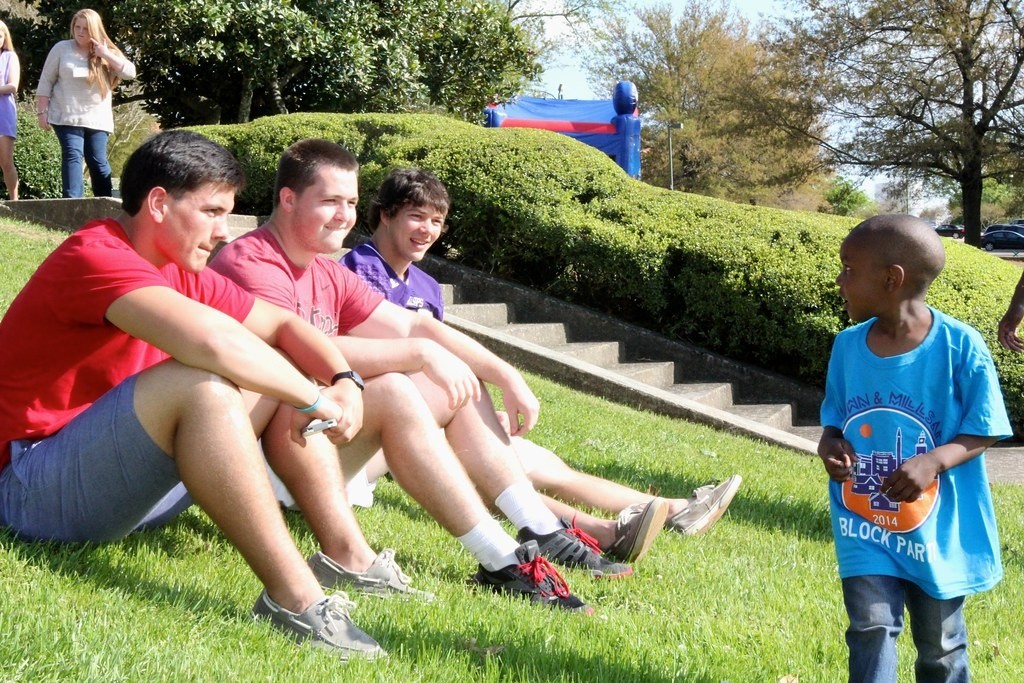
[331,370,366,390]
[295,397,321,412]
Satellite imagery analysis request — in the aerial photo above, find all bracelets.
[37,110,47,116]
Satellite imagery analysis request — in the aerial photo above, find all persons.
[36,9,137,198]
[818,214,1013,683]
[340,170,742,566]
[209,138,631,611]
[998,272,1024,353]
[0,130,436,660]
[0,20,20,201]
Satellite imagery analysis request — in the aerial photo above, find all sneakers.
[669,475,743,538]
[517,515,633,578]
[606,499,667,563]
[306,548,438,607]
[474,540,594,616]
[248,588,389,664]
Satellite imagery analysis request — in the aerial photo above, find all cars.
[980,230,1024,251]
[984,218,1024,235]
[934,224,964,238]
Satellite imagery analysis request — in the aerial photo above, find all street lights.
[667,122,684,191]
